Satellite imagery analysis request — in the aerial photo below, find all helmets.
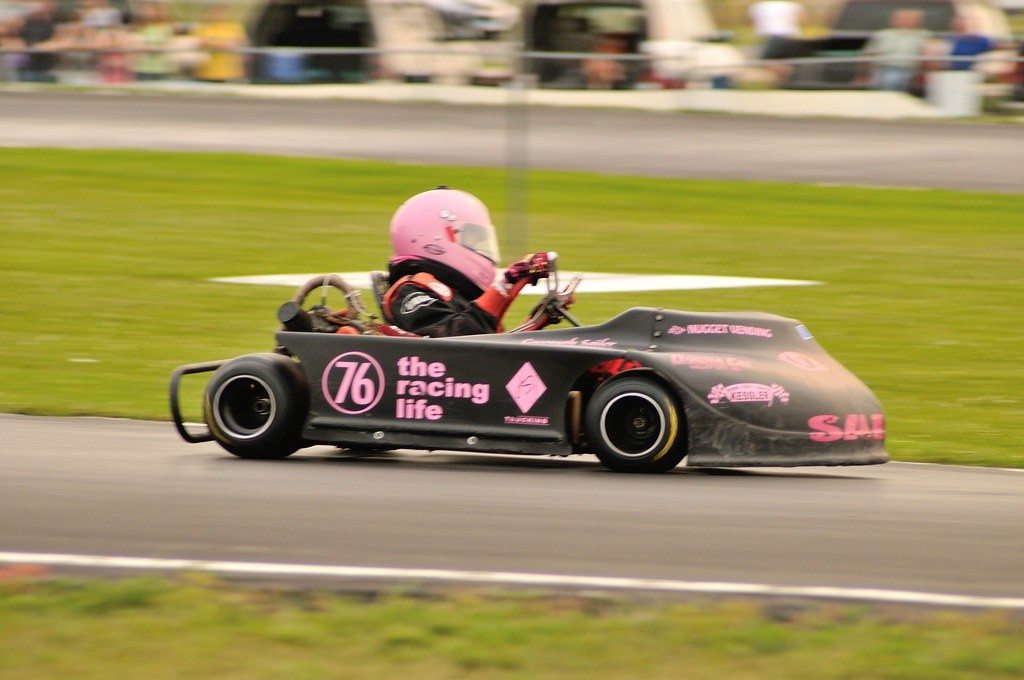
[390,188,496,299]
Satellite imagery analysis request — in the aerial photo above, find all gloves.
[532,292,573,328]
[504,252,558,288]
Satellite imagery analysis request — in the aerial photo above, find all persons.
[2,4,1014,108]
[377,187,578,339]
